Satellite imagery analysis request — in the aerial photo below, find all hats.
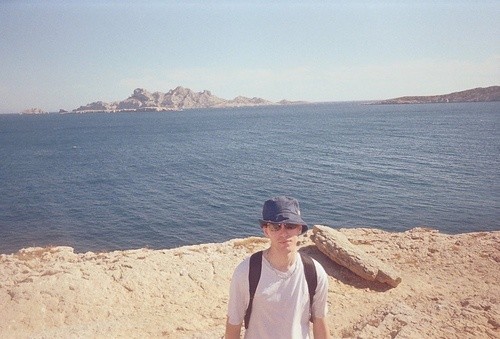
[257,195,309,237]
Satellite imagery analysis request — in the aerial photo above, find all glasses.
[265,223,300,231]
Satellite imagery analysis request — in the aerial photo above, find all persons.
[224,196,328,339]
